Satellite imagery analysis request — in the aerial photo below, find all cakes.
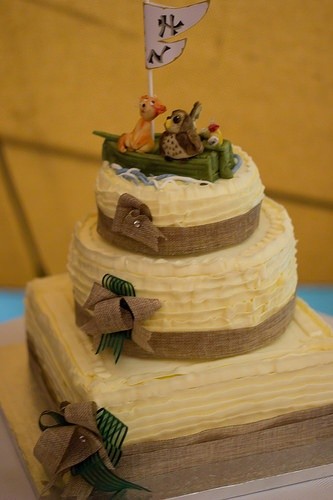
[24,132,332,500]
